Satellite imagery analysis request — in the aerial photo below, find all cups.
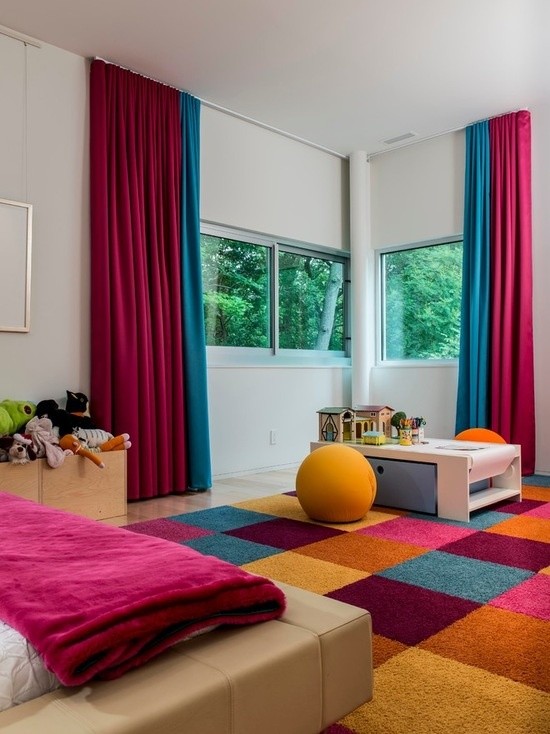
[400,430,413,445]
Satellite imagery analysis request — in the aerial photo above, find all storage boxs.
[0,449,127,523]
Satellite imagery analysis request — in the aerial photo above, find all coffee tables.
[310,437,521,522]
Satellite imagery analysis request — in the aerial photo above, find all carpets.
[119,476,549,734]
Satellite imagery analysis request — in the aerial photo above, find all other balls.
[295,443,377,523]
[455,427,508,444]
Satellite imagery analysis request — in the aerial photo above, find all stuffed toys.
[0,390,131,469]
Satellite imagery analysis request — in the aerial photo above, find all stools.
[0,493,373,733]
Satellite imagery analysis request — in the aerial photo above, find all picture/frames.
[0,198,34,332]
